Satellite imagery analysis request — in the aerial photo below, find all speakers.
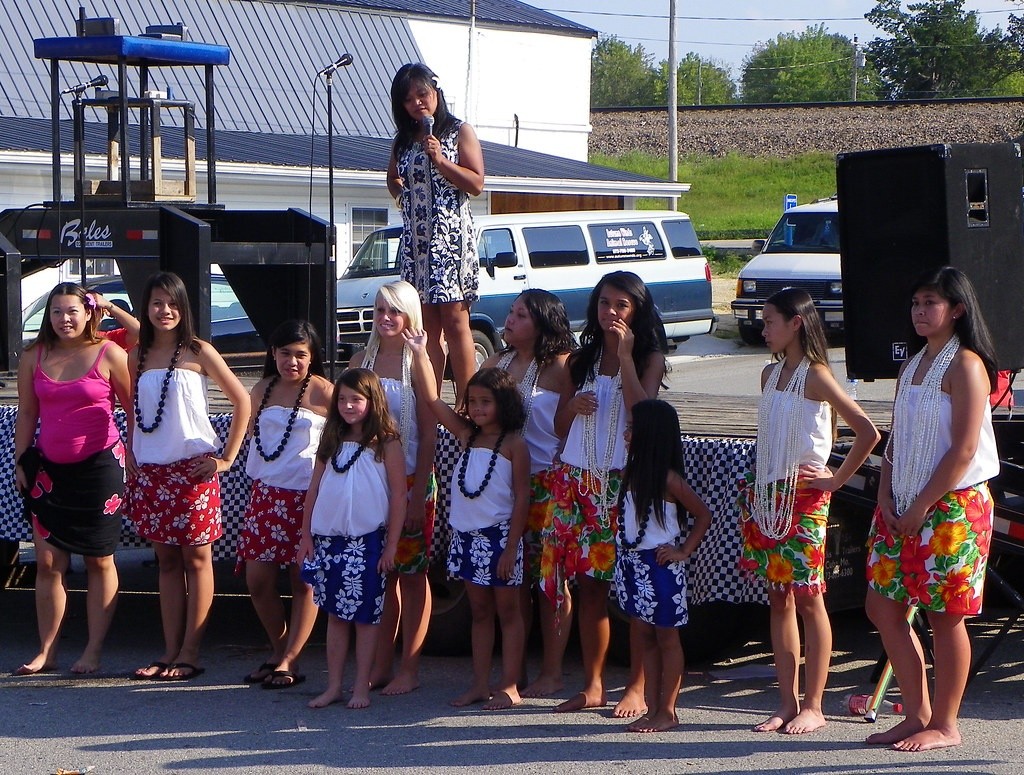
[837,140,1023,381]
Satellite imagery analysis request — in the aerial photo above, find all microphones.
[62,75,109,94]
[318,53,354,76]
[423,114,435,162]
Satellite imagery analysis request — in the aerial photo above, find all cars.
[21,272,269,354]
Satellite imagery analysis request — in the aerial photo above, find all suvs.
[731,199,845,349]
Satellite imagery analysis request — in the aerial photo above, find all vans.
[334,209,717,378]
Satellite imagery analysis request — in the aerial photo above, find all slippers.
[261,669,305,689]
[134,662,168,678]
[154,662,205,680]
[244,662,279,684]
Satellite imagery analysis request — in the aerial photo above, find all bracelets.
[395,193,407,210]
[108,304,114,311]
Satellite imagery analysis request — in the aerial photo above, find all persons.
[350,281,436,696]
[619,400,715,733]
[233,318,338,689]
[398,326,529,709]
[295,367,407,708]
[742,286,882,734]
[855,266,999,752]
[555,272,672,716]
[481,290,582,698]
[387,63,485,416]
[126,272,255,680]
[86,289,141,354]
[16,283,135,673]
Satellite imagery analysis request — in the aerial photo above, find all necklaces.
[358,334,412,458]
[614,473,651,549]
[253,368,310,462]
[577,344,627,529]
[490,339,554,439]
[743,354,811,541]
[331,427,377,473]
[458,428,504,500]
[884,329,960,519]
[133,337,183,433]
